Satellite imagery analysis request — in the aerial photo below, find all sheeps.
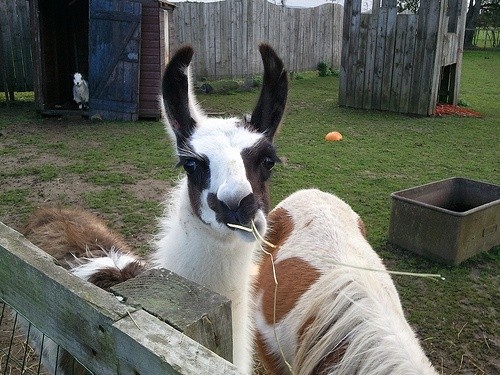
[252,188,442,375]
[11,43,289,375]
[71,72,89,111]
